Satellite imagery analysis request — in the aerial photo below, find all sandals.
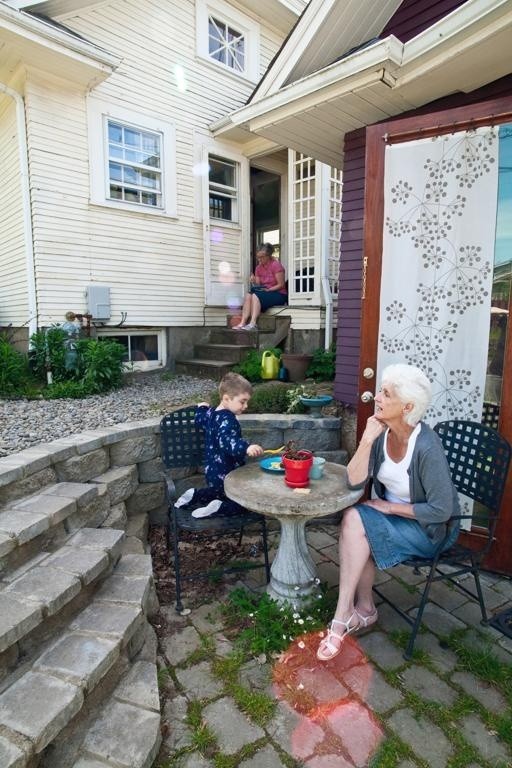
[317,609,364,661]
[354,608,379,630]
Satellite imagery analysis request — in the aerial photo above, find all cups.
[308,456,326,480]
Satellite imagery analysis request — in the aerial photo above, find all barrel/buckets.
[260,350,280,379]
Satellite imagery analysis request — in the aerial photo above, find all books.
[247,286,266,293]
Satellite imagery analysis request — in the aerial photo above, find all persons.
[173,371,263,518]
[317,365,462,662]
[231,242,288,330]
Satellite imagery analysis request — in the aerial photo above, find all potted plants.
[281,439,314,488]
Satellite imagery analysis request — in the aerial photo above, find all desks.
[223,454,370,621]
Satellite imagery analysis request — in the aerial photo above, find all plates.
[261,455,284,473]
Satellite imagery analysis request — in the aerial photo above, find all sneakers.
[174,487,195,509]
[240,323,259,331]
[232,322,246,330]
[191,499,223,519]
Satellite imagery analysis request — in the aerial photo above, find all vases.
[279,352,314,383]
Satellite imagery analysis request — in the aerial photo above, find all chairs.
[480,401,500,433]
[159,406,274,617]
[391,420,510,663]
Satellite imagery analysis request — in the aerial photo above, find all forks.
[249,445,285,456]
